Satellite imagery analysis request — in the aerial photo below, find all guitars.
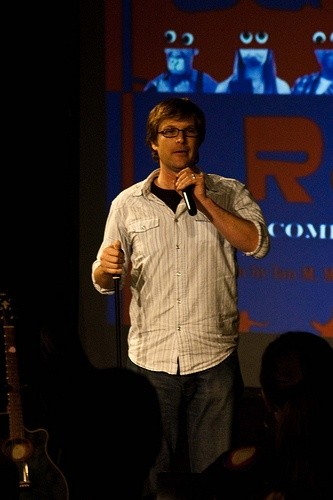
[0,293,70,500]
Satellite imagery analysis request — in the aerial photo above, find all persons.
[92,98,271,475]
[260,332,333,500]
[43,367,162,500]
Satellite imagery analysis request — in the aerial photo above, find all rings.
[192,174,196,180]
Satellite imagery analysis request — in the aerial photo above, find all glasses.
[156,128,200,138]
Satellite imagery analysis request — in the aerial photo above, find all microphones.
[176,177,197,216]
